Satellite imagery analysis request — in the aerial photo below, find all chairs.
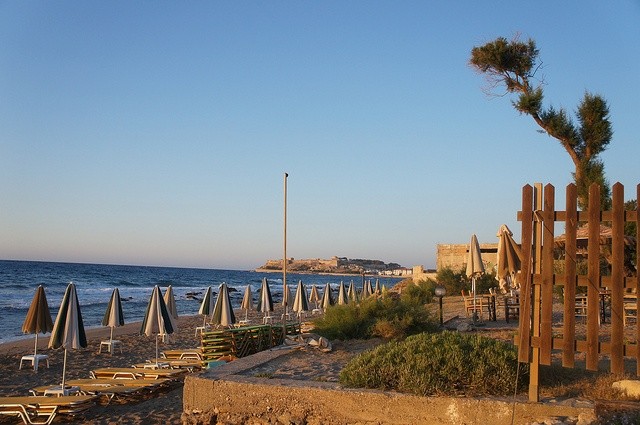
[622,286,638,326]
[462,291,483,319]
[199,322,302,369]
[560,286,587,321]
[0,348,203,425]
[503,296,520,322]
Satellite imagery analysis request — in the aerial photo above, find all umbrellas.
[309,284,322,309]
[163,286,178,341]
[140,285,177,365]
[282,284,292,314]
[292,280,308,334]
[496,224,522,316]
[256,277,274,325]
[211,282,236,331]
[338,281,347,305]
[303,284,308,318]
[363,280,368,298]
[368,280,373,295]
[466,234,485,313]
[22,285,54,356]
[375,279,380,293]
[318,282,335,312]
[241,284,253,320]
[198,286,214,327]
[102,288,124,340]
[348,280,357,303]
[48,282,88,389]
